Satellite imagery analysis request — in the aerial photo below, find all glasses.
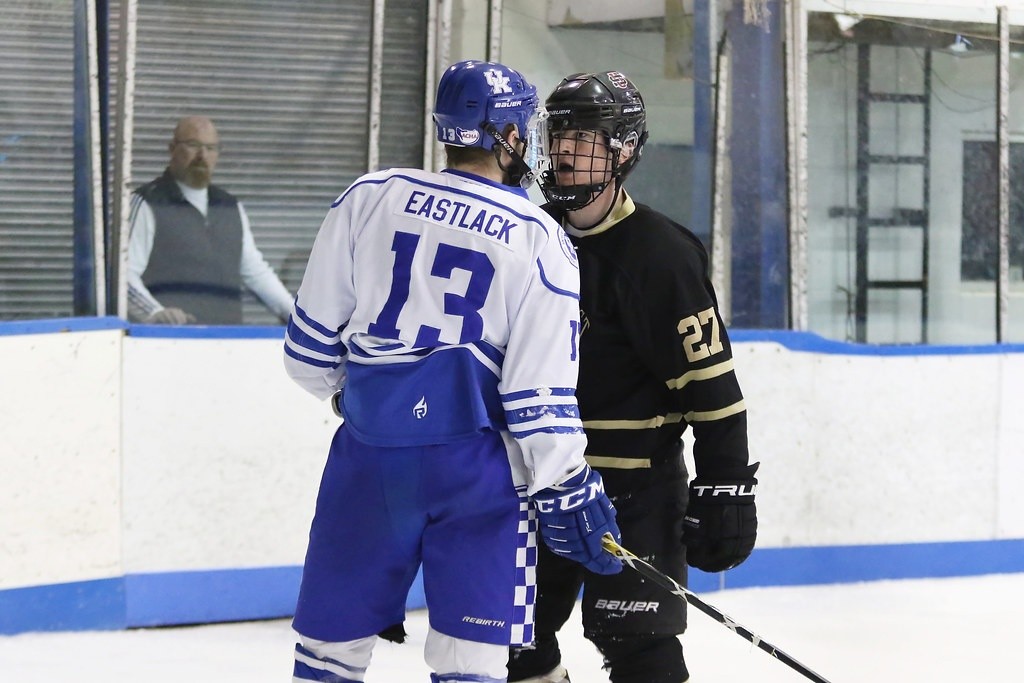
[178,139,220,154]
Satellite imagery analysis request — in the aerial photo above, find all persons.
[507,68,759,683]
[123,115,295,326]
[284,60,622,683]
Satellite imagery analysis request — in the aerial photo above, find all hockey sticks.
[601,537,832,683]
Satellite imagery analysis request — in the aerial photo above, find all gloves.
[532,458,624,575]
[679,462,761,572]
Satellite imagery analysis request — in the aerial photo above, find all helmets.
[536,68,646,211]
[433,58,539,153]
